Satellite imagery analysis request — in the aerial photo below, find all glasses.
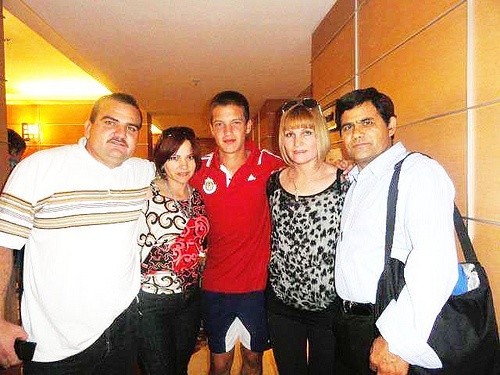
[281,98,323,117]
[162,126,194,137]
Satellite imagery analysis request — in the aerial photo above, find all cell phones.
[14,340,36,360]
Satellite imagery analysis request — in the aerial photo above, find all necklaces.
[164,180,192,217]
[291,177,308,201]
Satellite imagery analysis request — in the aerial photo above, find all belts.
[339,298,375,316]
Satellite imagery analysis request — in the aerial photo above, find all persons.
[266,98,352,375]
[6,128,26,170]
[335,87,458,375]
[189,91,354,375]
[0,93,156,375]
[140,126,209,375]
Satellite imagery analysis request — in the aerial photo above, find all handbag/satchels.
[375,152,500,374]
